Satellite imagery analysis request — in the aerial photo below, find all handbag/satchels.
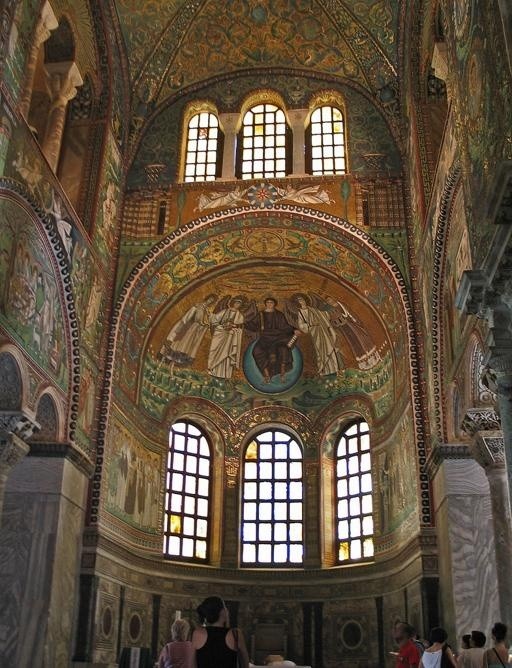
[440,644,456,667]
[235,648,245,667]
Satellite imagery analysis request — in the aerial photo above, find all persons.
[192,594,251,668]
[157,617,194,666]
[157,291,386,380]
[389,620,512,668]
